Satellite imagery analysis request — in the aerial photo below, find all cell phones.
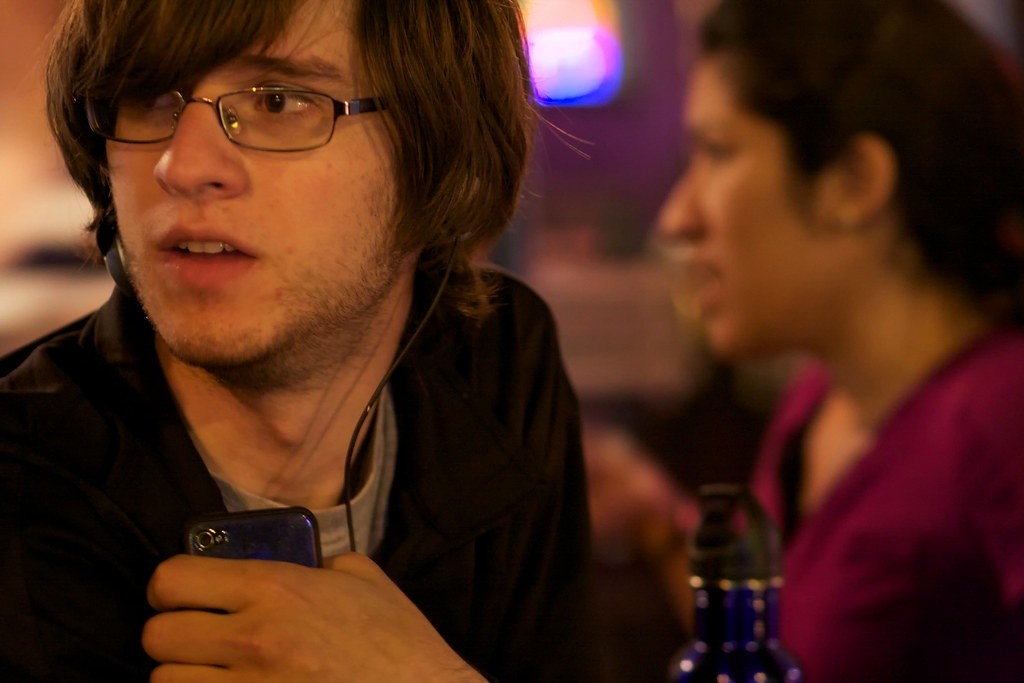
[186,506,322,613]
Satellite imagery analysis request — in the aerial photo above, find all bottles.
[667,484,805,683]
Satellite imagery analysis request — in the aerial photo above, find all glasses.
[71,78,386,154]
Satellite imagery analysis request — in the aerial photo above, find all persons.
[580,1,1024,683]
[0,1,597,683]
